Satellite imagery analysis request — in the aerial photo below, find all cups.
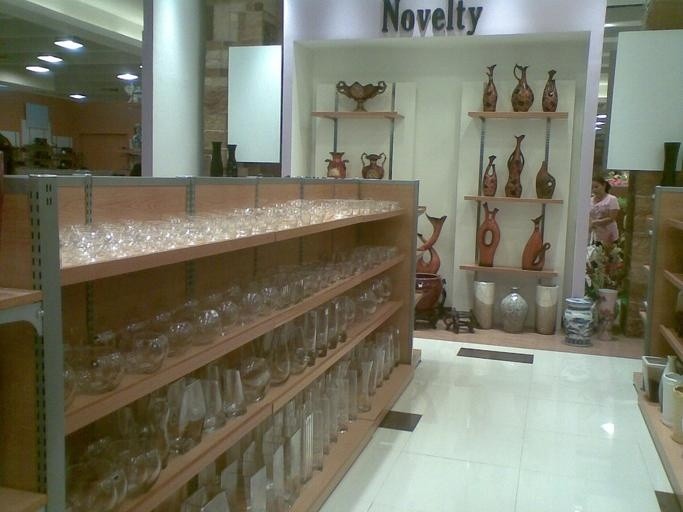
[662,371,683,445]
[641,355,669,403]
[65,240,403,512]
[60,197,404,269]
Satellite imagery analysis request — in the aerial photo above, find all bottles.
[657,354,678,415]
[209,141,238,177]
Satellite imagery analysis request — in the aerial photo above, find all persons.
[0,133,17,175]
[129,158,141,176]
[589,177,621,288]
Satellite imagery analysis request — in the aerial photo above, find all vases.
[210,141,236,177]
[413,272,618,348]
[642,353,683,444]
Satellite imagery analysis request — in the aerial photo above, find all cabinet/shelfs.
[633,185,683,512]
[0,168,419,511]
[458,110,567,279]
